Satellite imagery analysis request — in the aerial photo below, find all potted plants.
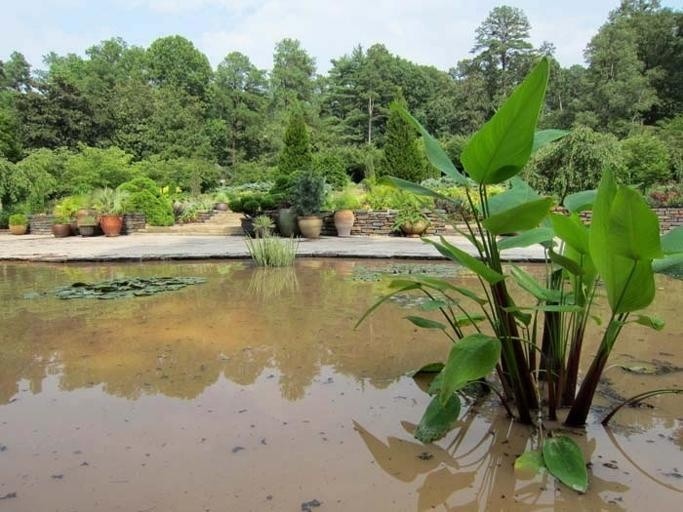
[215,193,230,211]
[8,184,130,236]
[228,168,354,239]
[391,206,432,238]
